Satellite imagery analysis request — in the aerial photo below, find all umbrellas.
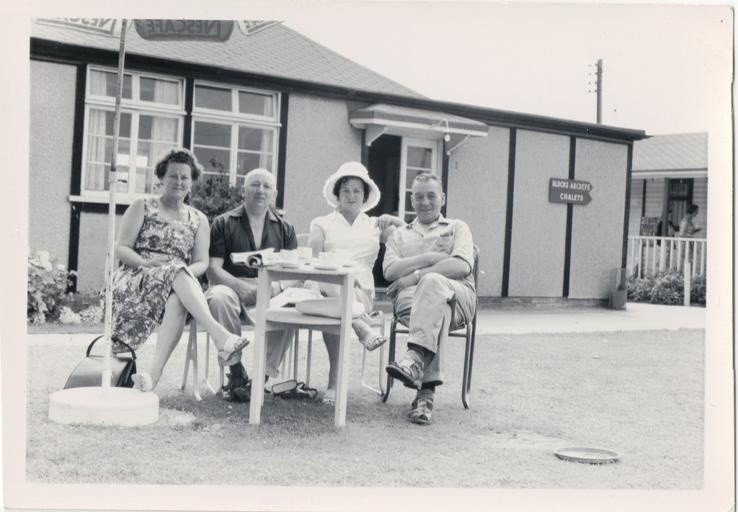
[32,16,284,388]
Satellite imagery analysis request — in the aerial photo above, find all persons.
[380,173,478,425]
[301,160,408,409]
[677,204,704,277]
[104,148,250,392]
[656,210,680,279]
[202,168,316,404]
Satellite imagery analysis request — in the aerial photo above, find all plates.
[276,260,300,269]
[312,264,339,271]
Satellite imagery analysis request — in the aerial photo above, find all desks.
[235,257,354,431]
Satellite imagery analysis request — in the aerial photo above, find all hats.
[322,161,382,214]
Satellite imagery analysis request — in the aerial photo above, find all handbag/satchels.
[64,334,136,389]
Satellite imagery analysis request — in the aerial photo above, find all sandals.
[359,335,386,351]
[386,350,426,390]
[408,390,434,425]
[323,390,335,407]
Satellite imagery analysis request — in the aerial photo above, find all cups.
[299,248,313,259]
[318,252,338,265]
[279,249,298,263]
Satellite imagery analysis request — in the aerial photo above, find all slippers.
[130,373,153,392]
[218,334,250,362]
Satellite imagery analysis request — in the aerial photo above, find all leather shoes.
[221,369,248,401]
[235,380,250,400]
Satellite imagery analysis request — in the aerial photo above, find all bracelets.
[414,271,420,280]
